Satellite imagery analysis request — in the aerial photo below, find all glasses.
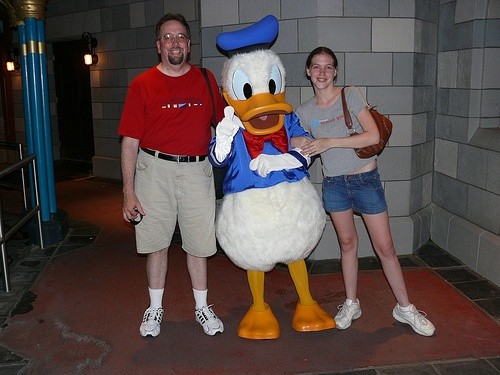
[159,33,189,44]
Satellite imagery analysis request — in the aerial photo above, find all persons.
[291,47,435,336]
[118,14,228,338]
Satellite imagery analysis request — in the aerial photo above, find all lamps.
[81,31,99,65]
[5,39,20,71]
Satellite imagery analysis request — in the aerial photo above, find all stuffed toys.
[208,14,337,340]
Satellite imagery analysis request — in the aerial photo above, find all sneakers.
[195,303,224,336]
[393,303,435,337]
[334,299,362,329]
[139,306,164,337]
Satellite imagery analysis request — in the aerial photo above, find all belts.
[140,148,207,162]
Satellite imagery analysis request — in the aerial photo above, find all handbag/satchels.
[341,85,393,158]
